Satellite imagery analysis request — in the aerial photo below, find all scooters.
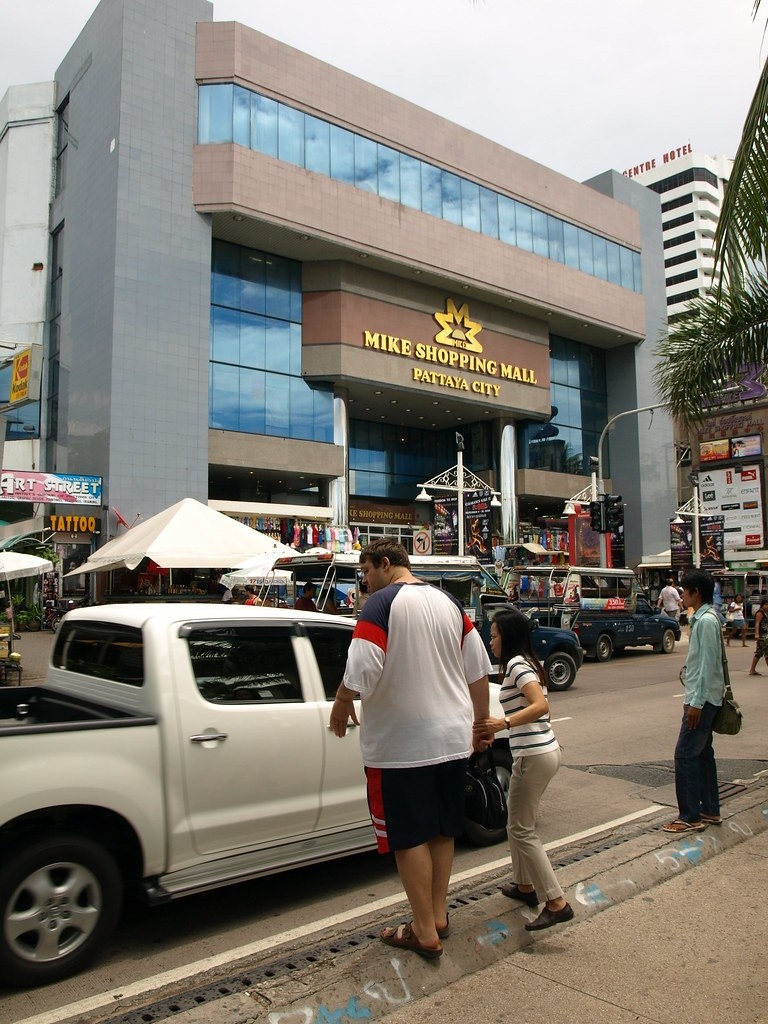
[47,592,91,633]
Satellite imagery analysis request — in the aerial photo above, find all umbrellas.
[0,551,54,633]
[62,497,305,595]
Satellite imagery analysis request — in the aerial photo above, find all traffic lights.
[605,494,624,531]
[590,501,606,534]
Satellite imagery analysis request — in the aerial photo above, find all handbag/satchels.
[725,603,735,622]
[462,745,508,828]
[711,697,741,735]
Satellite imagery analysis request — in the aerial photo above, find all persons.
[725,593,749,647]
[750,599,768,674]
[243,584,263,606]
[473,611,575,931]
[221,588,233,604]
[656,574,683,621]
[331,539,495,958]
[294,582,318,612]
[713,578,726,627]
[293,523,323,546]
[661,567,726,830]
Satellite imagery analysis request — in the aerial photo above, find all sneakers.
[501,881,539,907]
[526,903,573,930]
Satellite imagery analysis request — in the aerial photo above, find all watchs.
[504,716,510,730]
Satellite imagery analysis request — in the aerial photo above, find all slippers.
[700,813,723,824]
[749,672,761,675]
[662,820,705,832]
[436,912,452,937]
[380,920,444,958]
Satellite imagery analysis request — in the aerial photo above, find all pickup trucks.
[529,598,681,663]
[0,603,512,985]
[470,603,584,692]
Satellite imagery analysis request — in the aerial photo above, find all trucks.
[254,554,518,624]
[503,566,648,612]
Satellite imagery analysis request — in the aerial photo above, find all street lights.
[669,486,714,569]
[415,443,502,556]
[562,472,606,569]
